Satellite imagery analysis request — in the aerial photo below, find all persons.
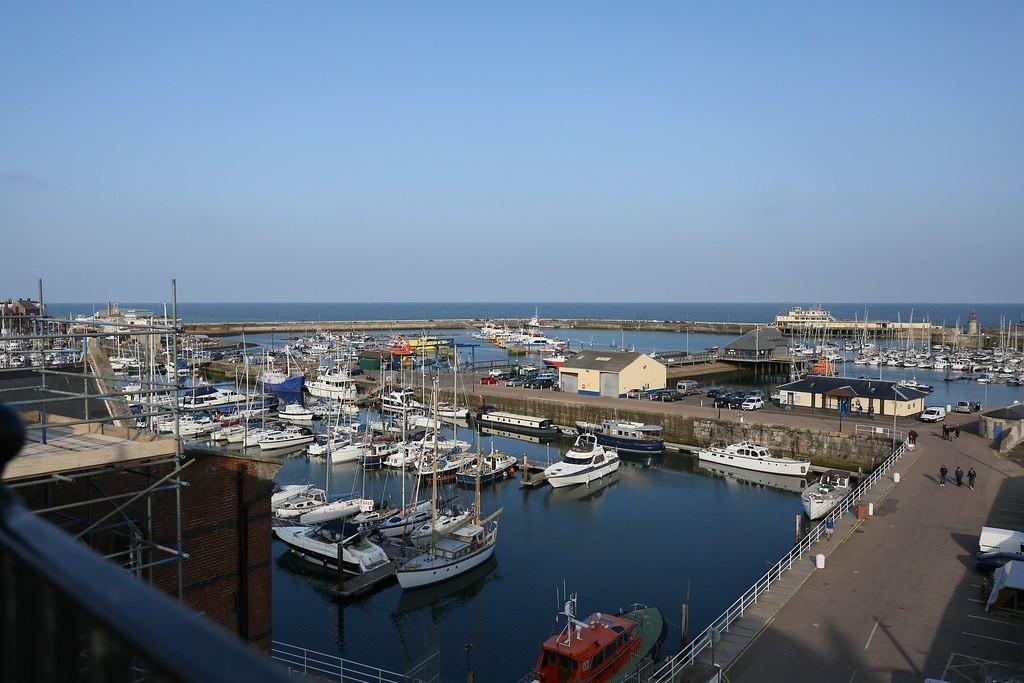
[868,404,875,417]
[967,467,976,491]
[940,465,948,487]
[826,515,834,541]
[955,466,963,487]
[942,422,960,442]
[912,431,919,445]
[857,403,863,415]
[909,430,914,444]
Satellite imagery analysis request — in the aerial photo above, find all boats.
[2,280,1023,683]
